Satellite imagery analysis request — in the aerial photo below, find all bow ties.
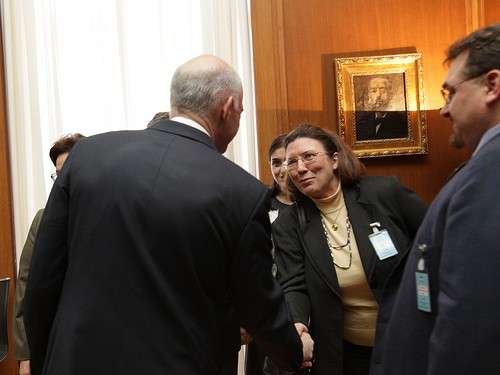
[375,117,385,126]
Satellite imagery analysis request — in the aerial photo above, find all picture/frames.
[335,52,429,157]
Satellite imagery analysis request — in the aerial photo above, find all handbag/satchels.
[263,356,315,375]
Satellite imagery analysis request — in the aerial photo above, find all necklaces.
[319,206,352,269]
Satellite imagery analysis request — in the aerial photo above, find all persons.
[12,133,90,375]
[244,133,298,375]
[377,23,500,375]
[356,74,409,140]
[272,124,432,375]
[16,52,314,375]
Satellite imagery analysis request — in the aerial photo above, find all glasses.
[282,150,330,171]
[51,172,60,182]
[441,72,486,104]
